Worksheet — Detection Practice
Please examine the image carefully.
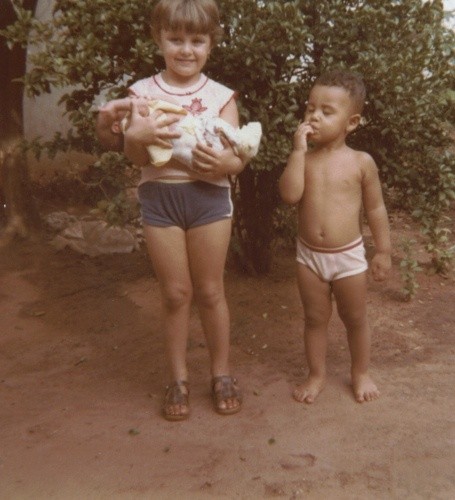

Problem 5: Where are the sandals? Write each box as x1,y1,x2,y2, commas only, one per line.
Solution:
211,375,244,415
163,379,190,420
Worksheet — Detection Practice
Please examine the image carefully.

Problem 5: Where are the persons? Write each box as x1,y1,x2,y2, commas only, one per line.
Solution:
277,68,394,405
124,0,245,423
95,86,262,183
